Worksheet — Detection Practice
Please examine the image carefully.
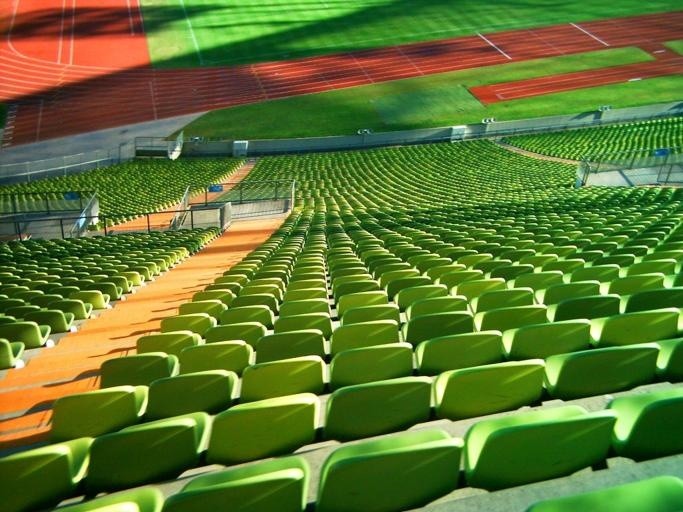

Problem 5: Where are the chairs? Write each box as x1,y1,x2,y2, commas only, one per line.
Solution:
0,114,683,511
